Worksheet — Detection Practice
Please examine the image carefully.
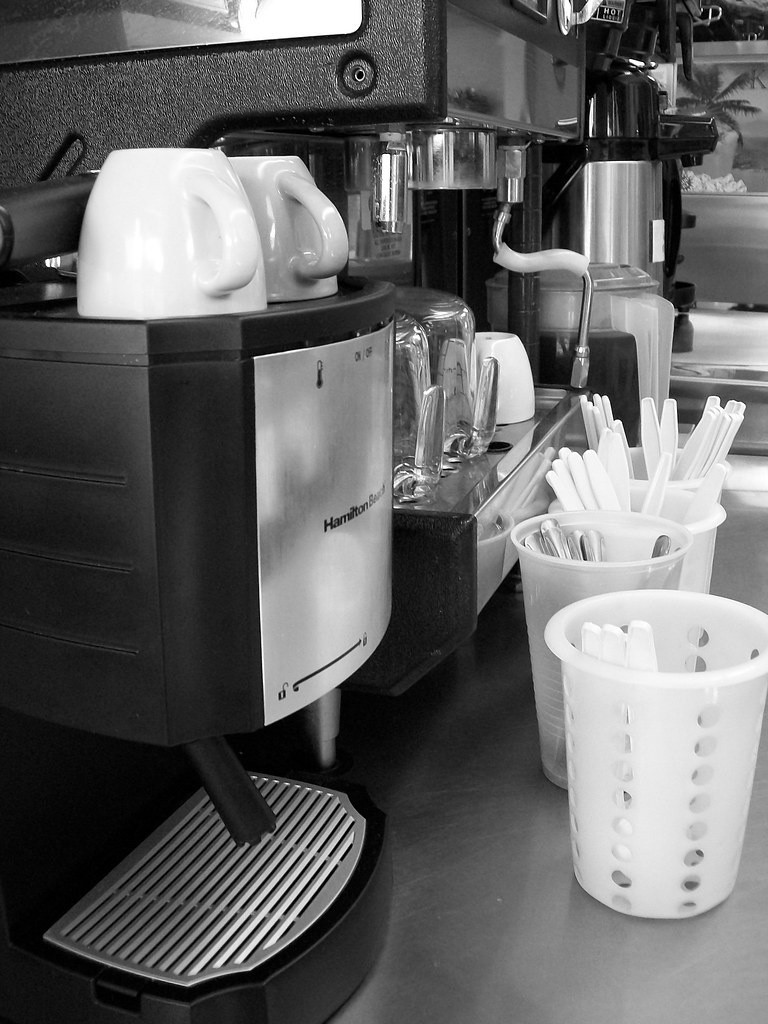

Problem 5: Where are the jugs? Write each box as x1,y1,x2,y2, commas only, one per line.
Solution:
486,263,675,447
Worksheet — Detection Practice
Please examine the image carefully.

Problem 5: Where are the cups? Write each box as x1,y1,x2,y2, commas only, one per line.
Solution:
76,148,268,323
600,446,732,508
393,311,445,503
228,156,349,303
546,488,729,594
510,509,694,791
473,331,536,424
544,589,768,920
395,289,498,463
